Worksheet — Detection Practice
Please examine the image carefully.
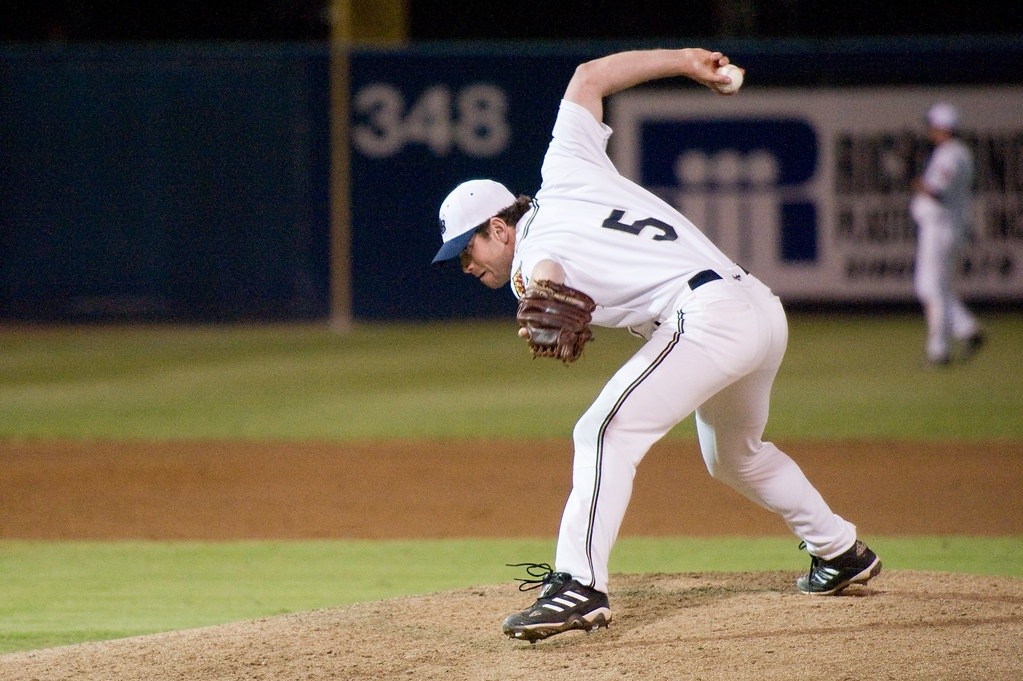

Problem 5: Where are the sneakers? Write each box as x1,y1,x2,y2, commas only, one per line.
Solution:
796,539,882,596
502,562,612,639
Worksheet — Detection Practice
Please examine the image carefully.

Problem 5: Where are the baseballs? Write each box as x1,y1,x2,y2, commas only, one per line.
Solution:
716,64,744,92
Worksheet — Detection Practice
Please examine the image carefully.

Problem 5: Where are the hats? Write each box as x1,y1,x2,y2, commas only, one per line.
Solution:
431,178,517,265
925,104,960,130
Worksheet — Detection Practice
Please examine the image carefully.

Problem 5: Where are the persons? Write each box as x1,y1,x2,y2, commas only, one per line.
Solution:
432,48,883,644
910,103,987,370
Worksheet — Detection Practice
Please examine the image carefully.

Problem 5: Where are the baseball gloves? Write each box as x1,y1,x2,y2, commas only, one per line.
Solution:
517,278,598,364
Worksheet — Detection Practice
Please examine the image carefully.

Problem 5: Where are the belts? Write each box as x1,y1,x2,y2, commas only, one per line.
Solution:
654,263,749,326
